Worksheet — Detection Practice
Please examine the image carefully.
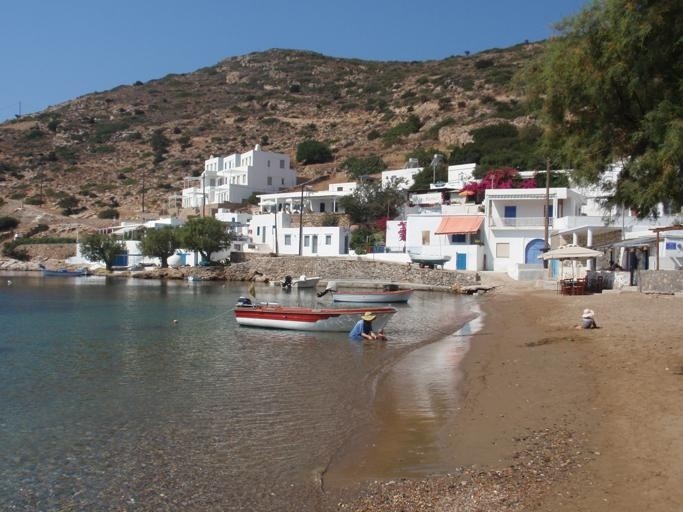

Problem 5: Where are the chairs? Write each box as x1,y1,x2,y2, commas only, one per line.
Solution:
560,276,603,295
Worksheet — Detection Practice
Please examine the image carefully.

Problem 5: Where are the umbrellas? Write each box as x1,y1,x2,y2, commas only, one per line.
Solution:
536,246,576,279
543,244,603,278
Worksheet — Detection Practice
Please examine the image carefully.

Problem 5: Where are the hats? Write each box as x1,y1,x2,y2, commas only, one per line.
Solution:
581,309,594,318
360,312,375,321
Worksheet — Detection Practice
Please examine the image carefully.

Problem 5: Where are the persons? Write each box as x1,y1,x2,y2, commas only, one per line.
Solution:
348,311,376,344
575,308,600,331
629,245,649,286
608,260,621,273
373,329,389,341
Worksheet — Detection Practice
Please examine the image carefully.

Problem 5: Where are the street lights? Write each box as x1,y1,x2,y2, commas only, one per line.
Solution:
298,183,313,257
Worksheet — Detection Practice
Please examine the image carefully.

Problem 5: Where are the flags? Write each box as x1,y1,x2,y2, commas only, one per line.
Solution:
248,281,255,297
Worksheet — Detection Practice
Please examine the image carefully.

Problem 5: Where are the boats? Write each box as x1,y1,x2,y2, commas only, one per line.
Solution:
409,250,451,270
41,268,93,277
184,274,218,282
233,294,397,337
268,275,321,289
317,281,415,305
